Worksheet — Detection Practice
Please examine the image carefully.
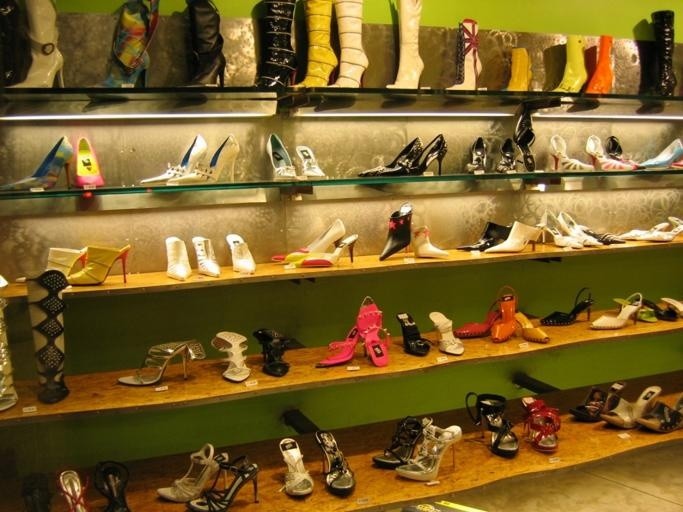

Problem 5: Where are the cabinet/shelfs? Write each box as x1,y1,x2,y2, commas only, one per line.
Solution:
0,82,683,510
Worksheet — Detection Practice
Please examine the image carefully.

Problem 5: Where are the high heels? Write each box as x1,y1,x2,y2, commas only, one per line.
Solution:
16,244,131,286
453,285,550,343
396,312,430,355
59,470,90,511
357,134,447,177
620,217,683,242
157,443,258,511
586,135,645,172
265,134,325,182
23,473,53,511
521,397,562,450
466,111,536,174
142,135,241,188
316,296,391,368
254,328,289,377
549,135,594,171
380,203,449,262
226,235,256,274
429,312,465,356
314,431,355,494
373,415,463,482
2,135,104,192
192,237,220,277
641,139,682,167
465,391,518,459
455,221,541,252
271,219,359,268
591,293,682,329
166,237,192,281
94,461,130,511
541,287,595,326
568,380,683,433
118,339,206,385
279,438,313,496
541,211,625,249
211,332,250,382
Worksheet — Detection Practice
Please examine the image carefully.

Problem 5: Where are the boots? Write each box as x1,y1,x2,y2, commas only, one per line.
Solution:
252,0,369,88
647,10,678,95
552,35,613,94
507,47,533,92
94,0,160,89
386,1,425,89
445,18,483,90
178,0,225,87
0,1,65,87
